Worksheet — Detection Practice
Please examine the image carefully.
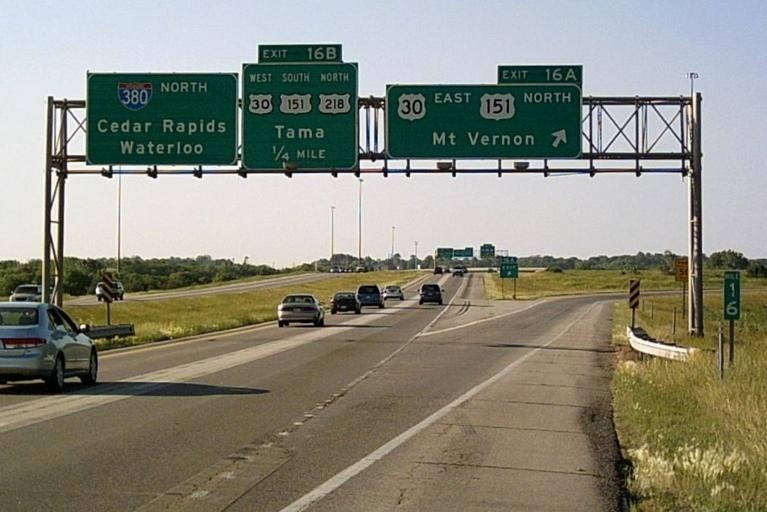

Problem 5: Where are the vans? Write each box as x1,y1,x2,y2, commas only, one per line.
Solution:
357,284,385,309
416,283,444,305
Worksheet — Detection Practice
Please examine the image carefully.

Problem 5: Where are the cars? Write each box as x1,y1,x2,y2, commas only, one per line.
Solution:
329,264,369,273
382,284,405,301
0,300,98,389
434,265,467,277
276,294,324,327
331,292,360,316
486,269,497,273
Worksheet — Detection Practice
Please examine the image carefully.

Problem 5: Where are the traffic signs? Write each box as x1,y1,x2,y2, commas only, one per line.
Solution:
84,71,240,167
436,248,452,258
257,43,342,64
240,64,358,170
385,84,582,157
454,250,465,256
500,263,518,278
503,257,518,262
496,63,582,86
674,257,689,281
480,246,494,258
464,247,472,256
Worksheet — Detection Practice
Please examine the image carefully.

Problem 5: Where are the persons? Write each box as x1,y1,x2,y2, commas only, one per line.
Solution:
18,310,35,325
53,317,66,332
304,298,312,303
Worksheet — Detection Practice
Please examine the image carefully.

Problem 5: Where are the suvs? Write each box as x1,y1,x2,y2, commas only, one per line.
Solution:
94,280,124,303
9,282,54,305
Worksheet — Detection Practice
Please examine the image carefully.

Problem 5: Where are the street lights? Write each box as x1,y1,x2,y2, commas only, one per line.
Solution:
357,178,364,265
390,226,396,259
331,206,337,264
414,241,418,269
